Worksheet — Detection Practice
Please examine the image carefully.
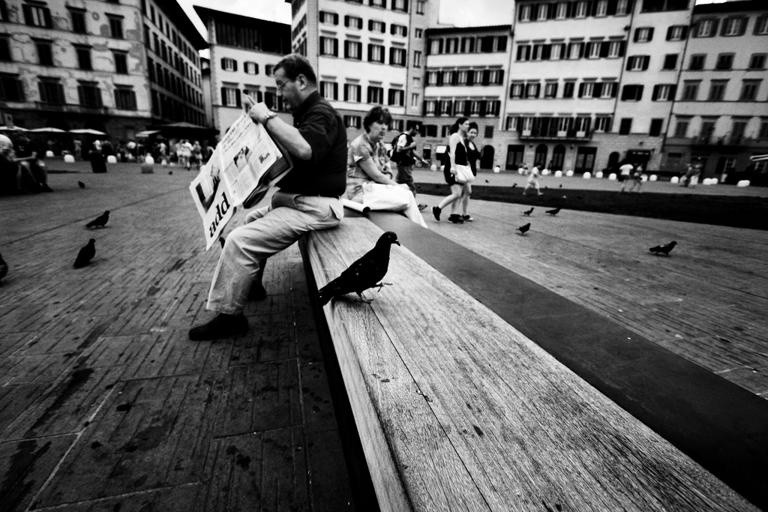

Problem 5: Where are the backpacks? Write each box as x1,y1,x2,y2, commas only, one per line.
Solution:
386,133,417,163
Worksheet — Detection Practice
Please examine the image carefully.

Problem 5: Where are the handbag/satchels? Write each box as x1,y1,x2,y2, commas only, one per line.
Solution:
453,163,475,184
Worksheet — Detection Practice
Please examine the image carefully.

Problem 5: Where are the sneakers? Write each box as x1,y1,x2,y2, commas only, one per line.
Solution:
417,203,471,224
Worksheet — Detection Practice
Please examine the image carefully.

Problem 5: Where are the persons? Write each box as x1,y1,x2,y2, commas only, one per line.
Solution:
629,162,644,193
521,162,544,196
618,161,634,195
187,52,346,346
344,104,429,229
431,115,469,226
1,106,204,189
462,121,486,224
389,120,431,213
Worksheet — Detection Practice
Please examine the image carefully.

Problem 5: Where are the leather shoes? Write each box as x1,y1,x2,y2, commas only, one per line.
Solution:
188,310,249,342
239,285,268,305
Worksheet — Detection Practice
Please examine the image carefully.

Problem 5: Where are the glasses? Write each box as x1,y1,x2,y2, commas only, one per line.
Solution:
276,79,293,92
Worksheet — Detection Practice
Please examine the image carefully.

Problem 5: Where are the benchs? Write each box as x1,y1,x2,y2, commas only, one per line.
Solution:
295,212,766,511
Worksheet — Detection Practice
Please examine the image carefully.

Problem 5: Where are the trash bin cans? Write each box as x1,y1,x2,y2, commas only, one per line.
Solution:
89,149,107,173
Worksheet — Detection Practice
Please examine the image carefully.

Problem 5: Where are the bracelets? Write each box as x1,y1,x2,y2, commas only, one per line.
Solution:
383,170,393,180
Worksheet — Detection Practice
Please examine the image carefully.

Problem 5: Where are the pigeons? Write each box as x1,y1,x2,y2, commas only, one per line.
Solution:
73,238,96,269
649,241,678,255
524,207,534,216
545,208,561,217
318,231,400,306
517,223,530,235
78,180,85,189
85,210,111,229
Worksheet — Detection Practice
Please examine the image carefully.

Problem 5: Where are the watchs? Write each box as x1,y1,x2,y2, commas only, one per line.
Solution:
262,110,278,127
